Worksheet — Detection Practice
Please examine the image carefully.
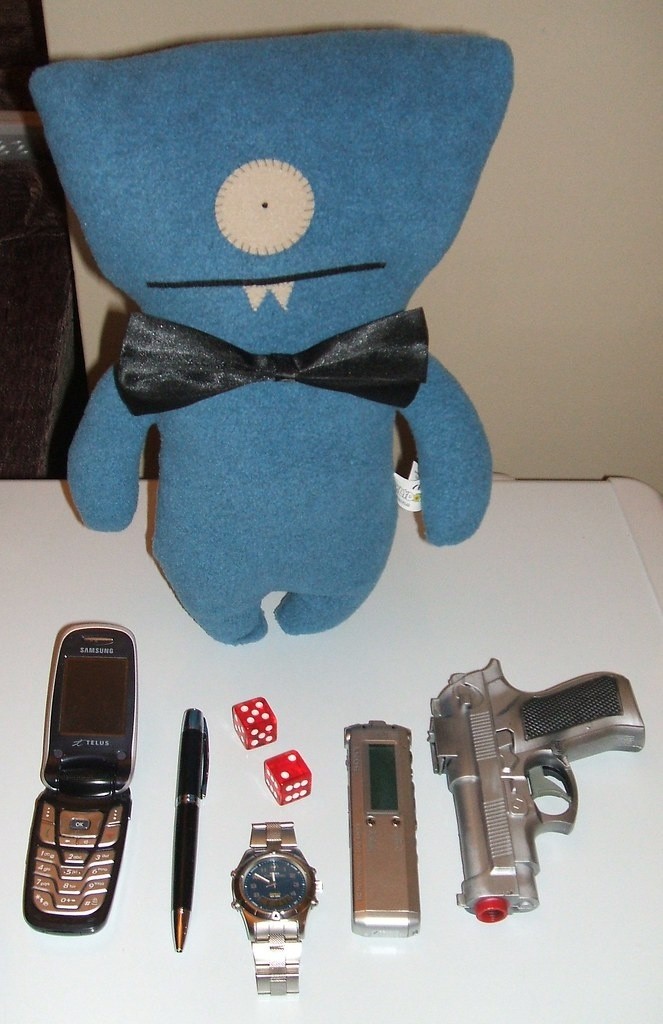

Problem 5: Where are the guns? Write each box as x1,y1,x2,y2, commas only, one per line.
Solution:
426,656,647,925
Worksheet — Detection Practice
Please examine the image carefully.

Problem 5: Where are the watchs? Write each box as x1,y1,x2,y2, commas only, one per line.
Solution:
231,822,324,997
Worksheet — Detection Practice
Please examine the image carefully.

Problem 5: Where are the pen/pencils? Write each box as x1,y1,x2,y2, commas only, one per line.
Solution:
172,710,212,954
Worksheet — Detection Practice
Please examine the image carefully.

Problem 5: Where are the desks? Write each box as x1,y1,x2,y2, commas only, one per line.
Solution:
0,471,663,1024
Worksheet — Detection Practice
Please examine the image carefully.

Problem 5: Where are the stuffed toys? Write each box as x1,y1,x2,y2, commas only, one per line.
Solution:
27,29,515,644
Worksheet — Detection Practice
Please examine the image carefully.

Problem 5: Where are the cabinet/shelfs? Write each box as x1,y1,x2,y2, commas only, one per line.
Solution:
0,0,86,474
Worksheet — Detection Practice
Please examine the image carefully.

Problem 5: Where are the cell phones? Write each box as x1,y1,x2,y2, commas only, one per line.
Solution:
22,623,137,935
